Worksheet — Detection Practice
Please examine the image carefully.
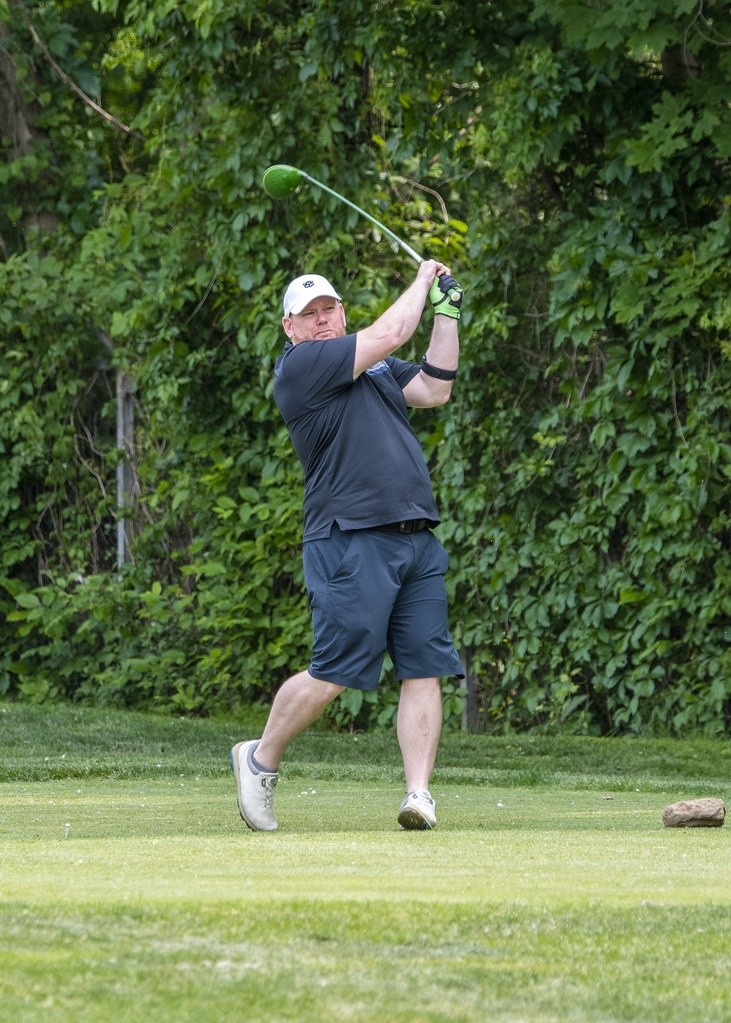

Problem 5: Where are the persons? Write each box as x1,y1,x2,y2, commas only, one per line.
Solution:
230,259,463,828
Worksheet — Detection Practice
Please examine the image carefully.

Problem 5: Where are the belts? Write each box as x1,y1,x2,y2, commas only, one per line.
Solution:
369,519,426,534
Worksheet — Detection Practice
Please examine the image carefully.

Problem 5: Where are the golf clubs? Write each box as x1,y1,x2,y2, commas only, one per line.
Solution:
261,163,462,303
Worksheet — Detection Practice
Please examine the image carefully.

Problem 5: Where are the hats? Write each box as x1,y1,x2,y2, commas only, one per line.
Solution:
283,274,342,317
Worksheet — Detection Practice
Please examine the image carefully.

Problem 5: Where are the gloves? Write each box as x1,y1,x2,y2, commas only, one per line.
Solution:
430,273,464,320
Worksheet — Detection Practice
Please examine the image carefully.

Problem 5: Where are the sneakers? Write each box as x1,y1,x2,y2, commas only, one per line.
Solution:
230,740,278,832
398,789,437,829
